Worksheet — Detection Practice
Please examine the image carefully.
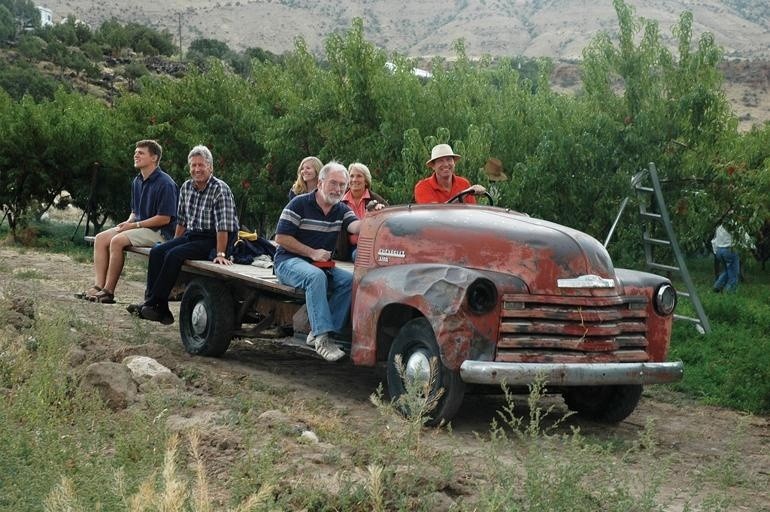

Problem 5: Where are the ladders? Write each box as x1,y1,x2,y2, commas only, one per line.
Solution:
634,162,711,335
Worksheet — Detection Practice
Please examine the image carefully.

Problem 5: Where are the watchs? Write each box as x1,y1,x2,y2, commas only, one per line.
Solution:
215,252,226,257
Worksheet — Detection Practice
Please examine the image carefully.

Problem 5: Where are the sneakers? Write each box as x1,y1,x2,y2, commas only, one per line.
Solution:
126,303,175,325
305,335,347,363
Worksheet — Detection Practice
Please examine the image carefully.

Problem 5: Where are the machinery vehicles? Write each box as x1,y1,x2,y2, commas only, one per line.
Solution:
83,191,684,427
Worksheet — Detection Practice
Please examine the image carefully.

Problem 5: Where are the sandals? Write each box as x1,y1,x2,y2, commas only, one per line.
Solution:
74,286,116,303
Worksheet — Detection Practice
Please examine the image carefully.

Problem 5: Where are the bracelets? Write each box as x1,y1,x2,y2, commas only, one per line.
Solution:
136,221,140,228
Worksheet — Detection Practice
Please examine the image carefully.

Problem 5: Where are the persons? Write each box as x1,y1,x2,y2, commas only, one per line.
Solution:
335,163,389,262
413,144,486,204
711,210,757,296
274,162,385,362
482,158,509,201
290,156,323,201
75,140,180,303
126,144,240,325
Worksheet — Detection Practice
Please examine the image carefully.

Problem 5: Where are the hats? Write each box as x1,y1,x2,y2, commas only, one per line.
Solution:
425,143,462,167
479,157,507,182
